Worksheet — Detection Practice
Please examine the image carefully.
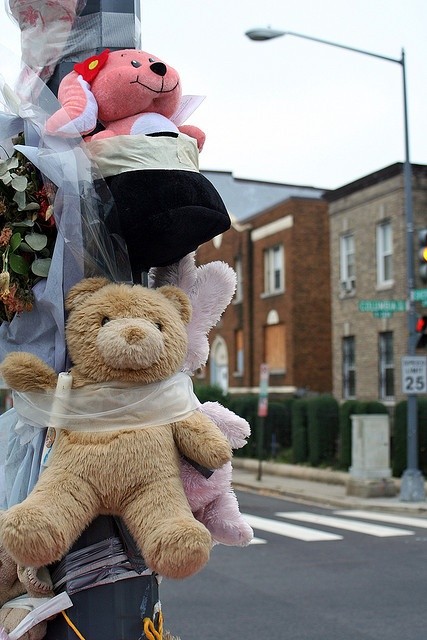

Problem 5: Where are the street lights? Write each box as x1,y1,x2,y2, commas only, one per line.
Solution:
246,28,427,503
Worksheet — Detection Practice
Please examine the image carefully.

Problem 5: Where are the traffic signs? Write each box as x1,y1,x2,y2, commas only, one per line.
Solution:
402,354,427,394
409,289,427,308
358,299,409,318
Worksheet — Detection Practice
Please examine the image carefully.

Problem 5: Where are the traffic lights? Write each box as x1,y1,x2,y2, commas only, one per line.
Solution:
417,229,427,281
416,314,427,349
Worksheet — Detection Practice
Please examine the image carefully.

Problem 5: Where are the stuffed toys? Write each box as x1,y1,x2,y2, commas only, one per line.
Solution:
0,544,53,640
44,49,232,266
0,276,234,579
144,249,255,548
